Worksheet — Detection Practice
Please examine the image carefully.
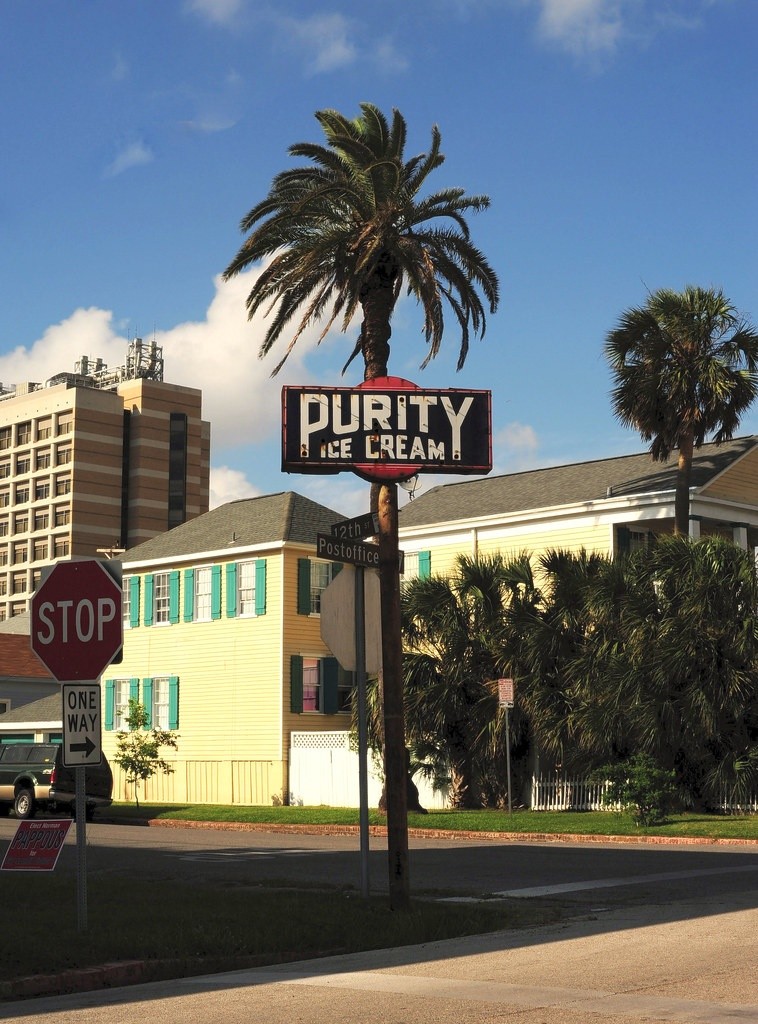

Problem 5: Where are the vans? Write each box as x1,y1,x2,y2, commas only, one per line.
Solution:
0,743,113,822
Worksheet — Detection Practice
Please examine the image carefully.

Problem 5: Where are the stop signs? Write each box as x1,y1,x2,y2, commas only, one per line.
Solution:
29,557,124,683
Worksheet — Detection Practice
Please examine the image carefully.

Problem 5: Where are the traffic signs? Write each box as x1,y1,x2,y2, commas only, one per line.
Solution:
63,680,102,767
